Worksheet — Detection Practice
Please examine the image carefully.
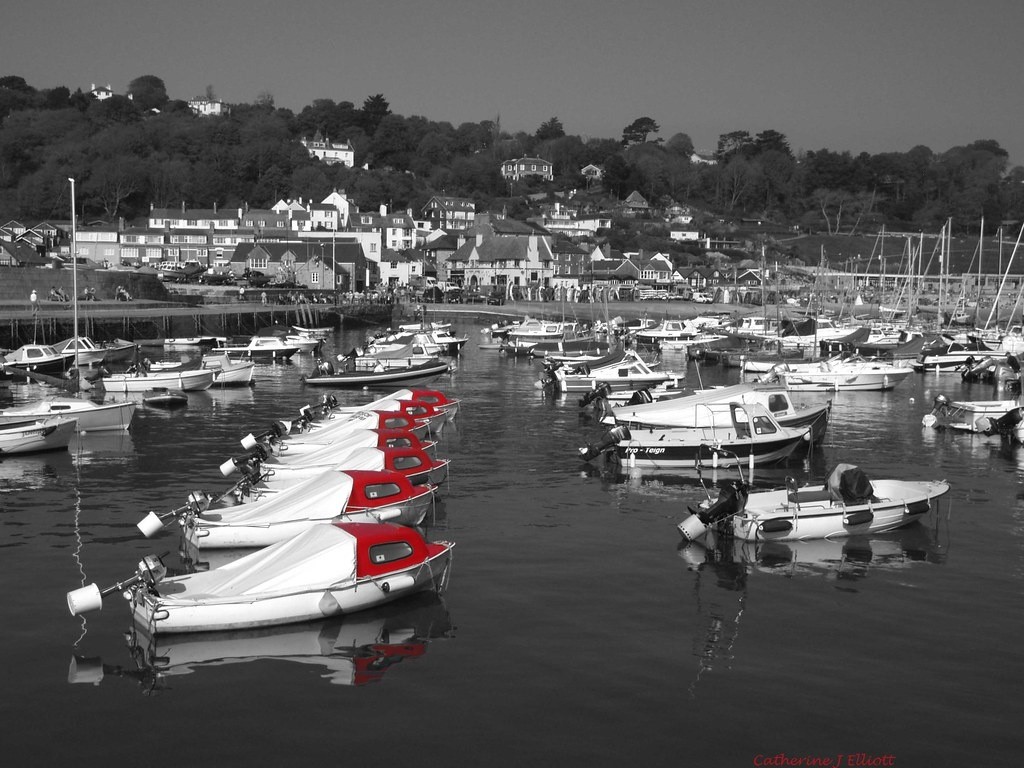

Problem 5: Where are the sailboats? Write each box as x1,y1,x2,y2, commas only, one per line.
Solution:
38,214,1024,407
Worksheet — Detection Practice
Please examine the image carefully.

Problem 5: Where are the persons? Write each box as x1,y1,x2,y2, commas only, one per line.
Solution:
48,284,133,304
236,278,413,306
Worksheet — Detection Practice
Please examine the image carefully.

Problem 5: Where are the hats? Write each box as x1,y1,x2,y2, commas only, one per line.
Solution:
1005,352,1010,356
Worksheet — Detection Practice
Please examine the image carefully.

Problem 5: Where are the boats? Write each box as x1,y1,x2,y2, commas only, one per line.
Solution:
611,382,832,446
64,521,457,638
134,467,438,551
218,386,463,482
916,340,1011,373
922,393,1024,433
578,400,811,467
1,342,74,379
677,442,951,543
0,394,139,432
1,414,77,453
974,406,1024,443
742,351,914,391
